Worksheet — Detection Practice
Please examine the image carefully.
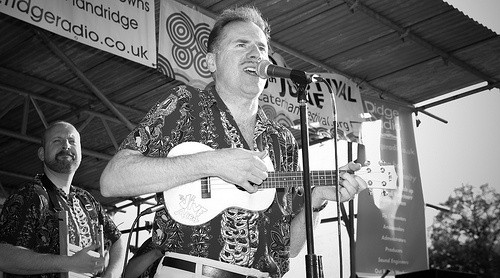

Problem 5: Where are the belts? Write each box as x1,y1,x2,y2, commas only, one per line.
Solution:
161,257,257,278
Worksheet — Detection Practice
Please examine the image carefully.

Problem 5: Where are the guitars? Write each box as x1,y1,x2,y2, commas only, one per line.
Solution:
163,141,399,226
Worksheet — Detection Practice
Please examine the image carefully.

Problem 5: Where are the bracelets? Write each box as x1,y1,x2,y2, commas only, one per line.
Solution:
311,186,328,212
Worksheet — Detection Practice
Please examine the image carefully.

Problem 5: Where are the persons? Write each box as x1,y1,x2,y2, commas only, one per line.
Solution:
0,122,126,278
100,7,368,278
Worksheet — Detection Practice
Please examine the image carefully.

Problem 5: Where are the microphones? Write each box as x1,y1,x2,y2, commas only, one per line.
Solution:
256,60,324,84
